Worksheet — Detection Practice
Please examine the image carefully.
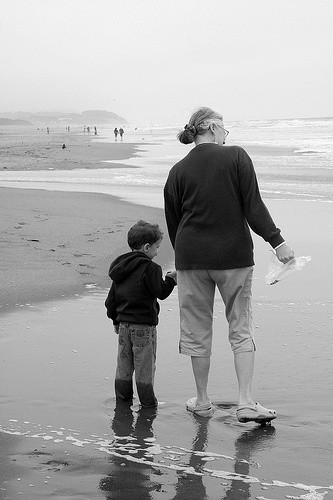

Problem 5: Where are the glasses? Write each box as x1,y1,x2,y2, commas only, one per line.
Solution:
217,125,229,137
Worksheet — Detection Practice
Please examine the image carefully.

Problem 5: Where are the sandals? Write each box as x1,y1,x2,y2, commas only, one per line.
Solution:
235,402,277,423
186,397,212,417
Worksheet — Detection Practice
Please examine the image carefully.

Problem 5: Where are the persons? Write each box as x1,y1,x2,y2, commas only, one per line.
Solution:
114,127,124,137
163,107,296,424
104,219,178,408
83,125,97,132
